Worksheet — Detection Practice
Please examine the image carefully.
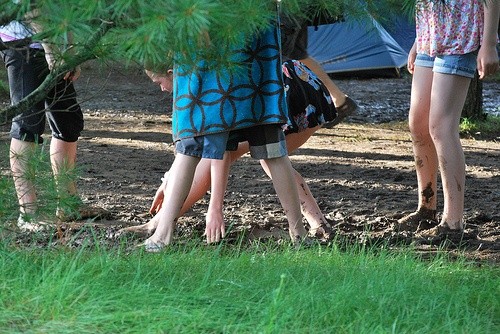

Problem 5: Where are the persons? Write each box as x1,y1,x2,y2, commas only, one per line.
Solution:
299,56,358,129
0,10,112,234
116,57,337,245
144,0,306,250
395,0,500,243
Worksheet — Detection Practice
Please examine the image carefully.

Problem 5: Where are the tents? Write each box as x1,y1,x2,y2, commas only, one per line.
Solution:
307,0,416,79
